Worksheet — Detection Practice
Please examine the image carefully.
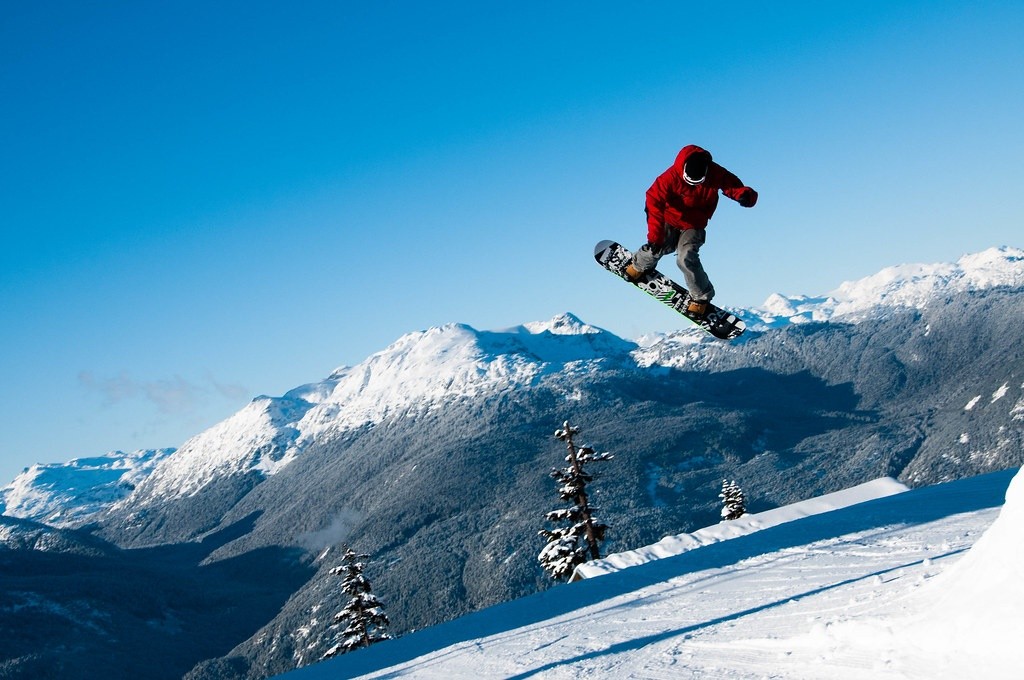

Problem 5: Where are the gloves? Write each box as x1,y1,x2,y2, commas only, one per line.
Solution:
739,191,750,207
650,243,660,255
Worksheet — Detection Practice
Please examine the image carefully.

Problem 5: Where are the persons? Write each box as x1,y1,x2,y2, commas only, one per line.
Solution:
622,143,759,319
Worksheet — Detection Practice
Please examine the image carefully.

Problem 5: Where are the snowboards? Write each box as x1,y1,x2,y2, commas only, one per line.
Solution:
595,238,747,340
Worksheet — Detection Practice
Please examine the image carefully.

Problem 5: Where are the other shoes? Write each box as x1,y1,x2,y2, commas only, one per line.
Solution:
624,265,642,280
688,300,708,315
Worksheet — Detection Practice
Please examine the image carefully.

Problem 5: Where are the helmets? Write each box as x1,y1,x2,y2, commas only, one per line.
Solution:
683,153,710,185
